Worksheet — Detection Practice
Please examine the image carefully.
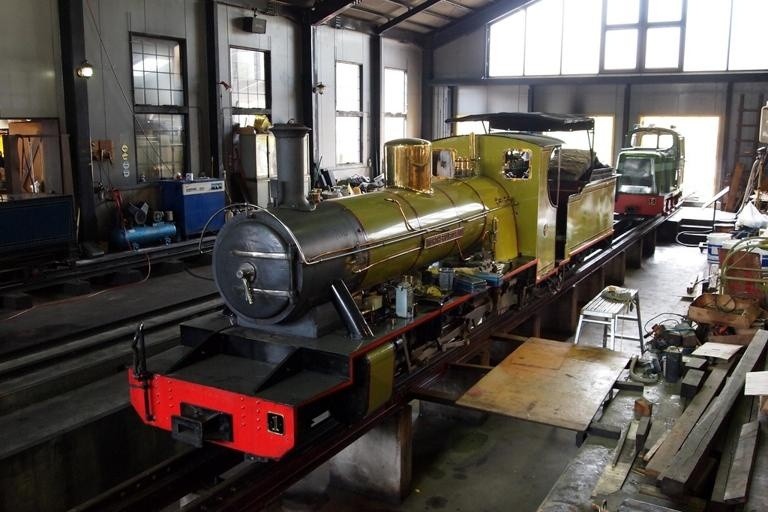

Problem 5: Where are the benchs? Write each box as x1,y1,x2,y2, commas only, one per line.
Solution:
573,284,644,355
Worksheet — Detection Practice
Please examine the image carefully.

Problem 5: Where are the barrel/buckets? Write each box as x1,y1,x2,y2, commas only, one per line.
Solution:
755,248,768,270
396,284,413,317
439,267,454,290
706,232,731,264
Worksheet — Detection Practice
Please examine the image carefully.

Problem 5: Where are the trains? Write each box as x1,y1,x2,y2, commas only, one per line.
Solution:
128,111,685,480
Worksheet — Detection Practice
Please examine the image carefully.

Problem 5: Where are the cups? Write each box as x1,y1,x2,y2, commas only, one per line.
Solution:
440,267,455,292
186,173,193,181
